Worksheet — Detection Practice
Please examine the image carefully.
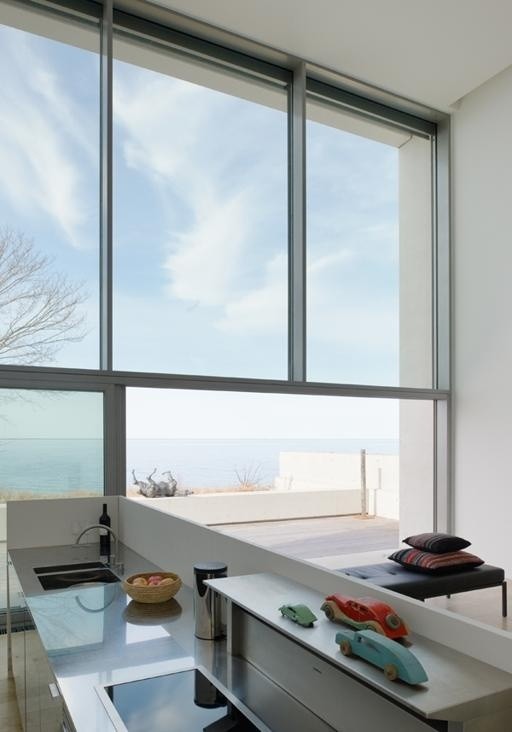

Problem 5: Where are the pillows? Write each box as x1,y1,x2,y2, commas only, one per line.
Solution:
387,529,482,575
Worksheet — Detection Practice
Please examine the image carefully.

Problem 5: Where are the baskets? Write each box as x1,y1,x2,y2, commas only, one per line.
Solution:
124,573,182,603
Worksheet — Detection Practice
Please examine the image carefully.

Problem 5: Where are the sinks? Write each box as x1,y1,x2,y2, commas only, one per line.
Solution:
32,561,110,575
36,567,122,591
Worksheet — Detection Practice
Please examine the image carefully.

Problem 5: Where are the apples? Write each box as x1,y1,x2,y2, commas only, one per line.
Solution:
132,575,174,586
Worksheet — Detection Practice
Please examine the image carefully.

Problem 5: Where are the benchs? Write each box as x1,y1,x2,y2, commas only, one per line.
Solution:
332,558,507,620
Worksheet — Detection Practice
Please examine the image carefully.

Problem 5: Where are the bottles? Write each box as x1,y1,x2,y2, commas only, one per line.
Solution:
192,561,229,642
99,503,111,555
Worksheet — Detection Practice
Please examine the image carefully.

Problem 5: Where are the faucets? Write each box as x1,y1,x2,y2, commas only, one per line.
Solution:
75,585,121,613
74,523,119,566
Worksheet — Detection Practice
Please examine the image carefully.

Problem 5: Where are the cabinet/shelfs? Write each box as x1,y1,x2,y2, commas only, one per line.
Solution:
6,552,63,731
